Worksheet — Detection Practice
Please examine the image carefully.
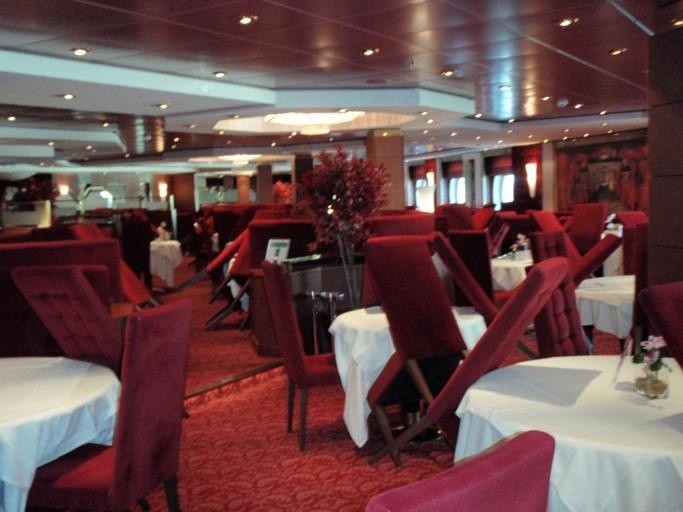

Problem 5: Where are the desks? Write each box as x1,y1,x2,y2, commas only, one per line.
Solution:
454,356,683,511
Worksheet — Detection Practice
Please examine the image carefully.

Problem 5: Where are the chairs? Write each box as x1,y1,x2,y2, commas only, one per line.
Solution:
0,208,192,512
193,204,682,469
364,430,555,511
